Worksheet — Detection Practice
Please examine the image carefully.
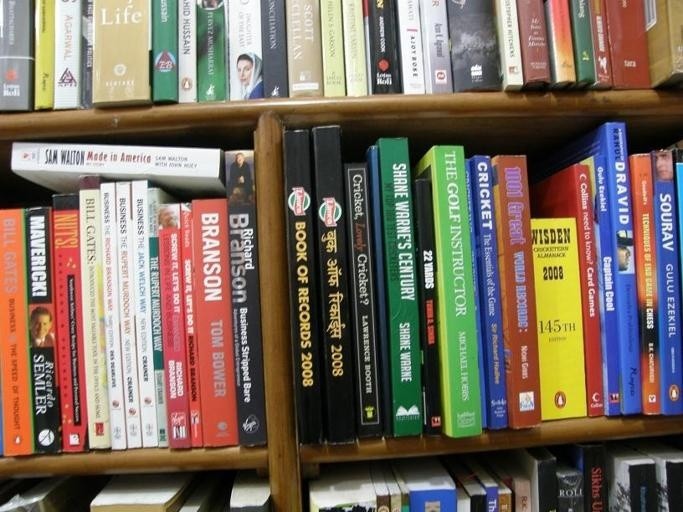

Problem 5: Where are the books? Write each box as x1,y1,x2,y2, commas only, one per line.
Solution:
0,0,683,113
309,436,683,512
1,142,267,456
0,474,272,512
283,121,683,446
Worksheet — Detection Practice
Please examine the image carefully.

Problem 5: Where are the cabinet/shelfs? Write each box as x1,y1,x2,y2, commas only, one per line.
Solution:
0,86,683,512
